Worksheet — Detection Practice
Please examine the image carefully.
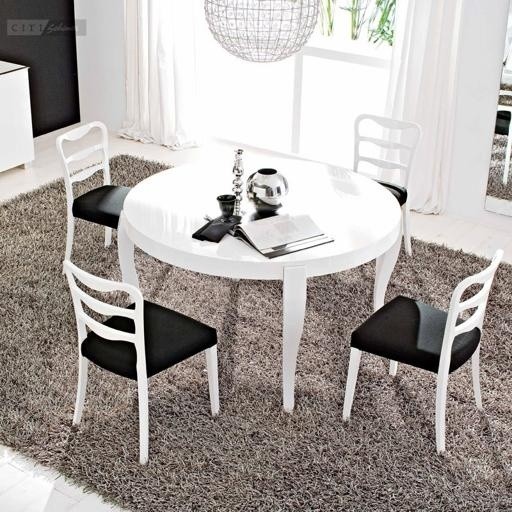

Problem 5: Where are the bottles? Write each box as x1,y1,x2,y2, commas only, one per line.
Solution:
246,168,289,212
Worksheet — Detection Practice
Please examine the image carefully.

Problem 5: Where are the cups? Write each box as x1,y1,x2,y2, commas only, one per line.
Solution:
216,194,236,222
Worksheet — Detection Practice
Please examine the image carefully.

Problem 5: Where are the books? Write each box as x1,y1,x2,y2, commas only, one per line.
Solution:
203,204,336,259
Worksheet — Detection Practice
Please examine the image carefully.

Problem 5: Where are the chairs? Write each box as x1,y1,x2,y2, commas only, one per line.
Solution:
353,112,421,259
62,261,221,465
343,249,505,454
56,118,139,277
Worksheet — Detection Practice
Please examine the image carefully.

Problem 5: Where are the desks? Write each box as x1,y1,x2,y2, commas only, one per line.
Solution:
117,147,403,414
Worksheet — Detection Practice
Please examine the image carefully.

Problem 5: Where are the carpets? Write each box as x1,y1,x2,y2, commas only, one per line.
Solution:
0,150,512,510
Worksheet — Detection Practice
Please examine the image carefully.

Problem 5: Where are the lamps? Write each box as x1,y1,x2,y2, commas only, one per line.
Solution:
204,1,320,64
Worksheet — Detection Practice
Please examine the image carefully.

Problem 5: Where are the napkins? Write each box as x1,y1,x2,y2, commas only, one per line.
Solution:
191,215,242,243
